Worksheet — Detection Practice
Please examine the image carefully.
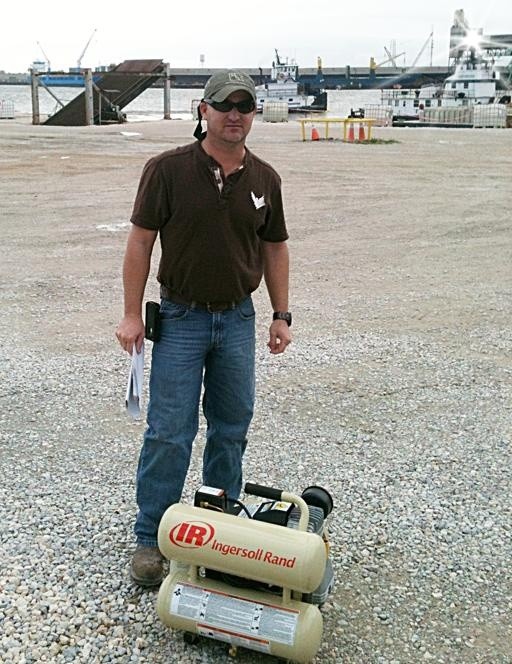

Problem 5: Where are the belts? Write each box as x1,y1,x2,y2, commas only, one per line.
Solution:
160,285,240,313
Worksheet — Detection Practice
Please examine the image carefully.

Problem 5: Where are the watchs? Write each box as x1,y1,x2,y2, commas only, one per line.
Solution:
273,312,291,327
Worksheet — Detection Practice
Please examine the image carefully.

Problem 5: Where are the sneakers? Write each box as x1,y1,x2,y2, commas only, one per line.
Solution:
128,544,164,586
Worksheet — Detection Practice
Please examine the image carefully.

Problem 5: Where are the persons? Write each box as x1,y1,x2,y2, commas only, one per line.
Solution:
116,70,292,587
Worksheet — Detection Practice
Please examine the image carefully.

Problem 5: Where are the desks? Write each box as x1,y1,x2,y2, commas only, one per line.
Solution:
296,117,376,145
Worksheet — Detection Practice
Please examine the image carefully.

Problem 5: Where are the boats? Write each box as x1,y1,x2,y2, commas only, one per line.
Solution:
255,57,301,112
330,46,512,127
33,29,116,86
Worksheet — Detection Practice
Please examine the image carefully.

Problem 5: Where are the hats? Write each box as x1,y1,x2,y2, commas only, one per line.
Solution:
204,68,256,105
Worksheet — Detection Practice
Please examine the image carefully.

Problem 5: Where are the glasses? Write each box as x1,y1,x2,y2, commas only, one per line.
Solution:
203,99,256,113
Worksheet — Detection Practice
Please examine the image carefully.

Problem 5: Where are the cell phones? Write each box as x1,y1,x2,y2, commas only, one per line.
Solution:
147,301,161,337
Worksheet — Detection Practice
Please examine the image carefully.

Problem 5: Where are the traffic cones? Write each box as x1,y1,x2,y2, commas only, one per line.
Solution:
310,122,319,141
347,116,367,142
